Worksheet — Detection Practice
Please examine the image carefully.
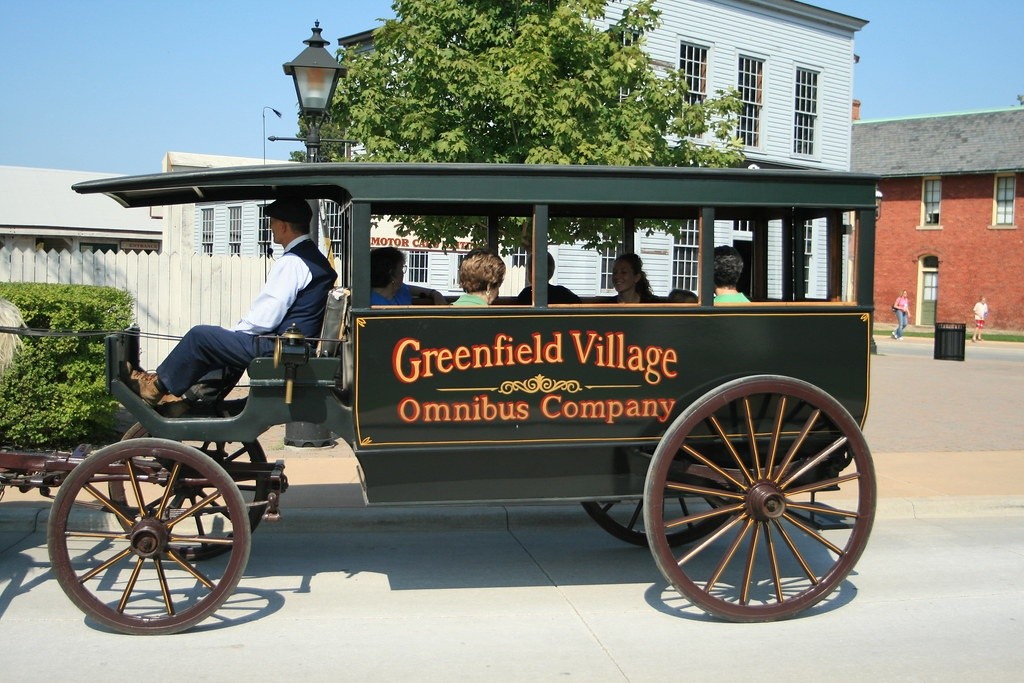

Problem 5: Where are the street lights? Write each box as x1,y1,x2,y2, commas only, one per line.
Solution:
281,16,351,253
260,105,283,286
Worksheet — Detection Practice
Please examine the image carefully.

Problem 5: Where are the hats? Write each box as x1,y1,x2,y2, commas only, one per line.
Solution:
264,198,313,223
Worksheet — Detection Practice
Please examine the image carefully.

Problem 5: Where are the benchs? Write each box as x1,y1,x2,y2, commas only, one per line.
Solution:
249,284,350,379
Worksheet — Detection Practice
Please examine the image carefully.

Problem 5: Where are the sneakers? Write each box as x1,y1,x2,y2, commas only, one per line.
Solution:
121,360,166,409
156,394,192,417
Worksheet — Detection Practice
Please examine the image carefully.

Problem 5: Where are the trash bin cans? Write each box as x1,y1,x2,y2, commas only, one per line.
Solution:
283,420,337,448
934,322,965,362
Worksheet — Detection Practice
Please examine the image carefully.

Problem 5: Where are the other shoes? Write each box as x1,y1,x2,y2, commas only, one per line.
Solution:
892,332,898,338
978,338,982,340
972,339,977,343
899,337,904,341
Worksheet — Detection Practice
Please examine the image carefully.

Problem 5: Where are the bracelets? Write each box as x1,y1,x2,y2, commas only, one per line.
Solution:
428,290,435,297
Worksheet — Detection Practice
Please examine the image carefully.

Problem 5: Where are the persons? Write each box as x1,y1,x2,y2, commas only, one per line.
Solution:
370,247,447,306
713,245,751,303
667,289,697,303
972,296,989,341
891,289,911,339
598,253,666,303
120,199,339,418
448,247,506,305
517,252,582,305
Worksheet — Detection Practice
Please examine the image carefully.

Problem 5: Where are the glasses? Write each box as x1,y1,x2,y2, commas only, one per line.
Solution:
401,266,407,274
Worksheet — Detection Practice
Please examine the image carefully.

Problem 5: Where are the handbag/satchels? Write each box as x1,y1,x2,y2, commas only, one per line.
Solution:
892,295,902,312
